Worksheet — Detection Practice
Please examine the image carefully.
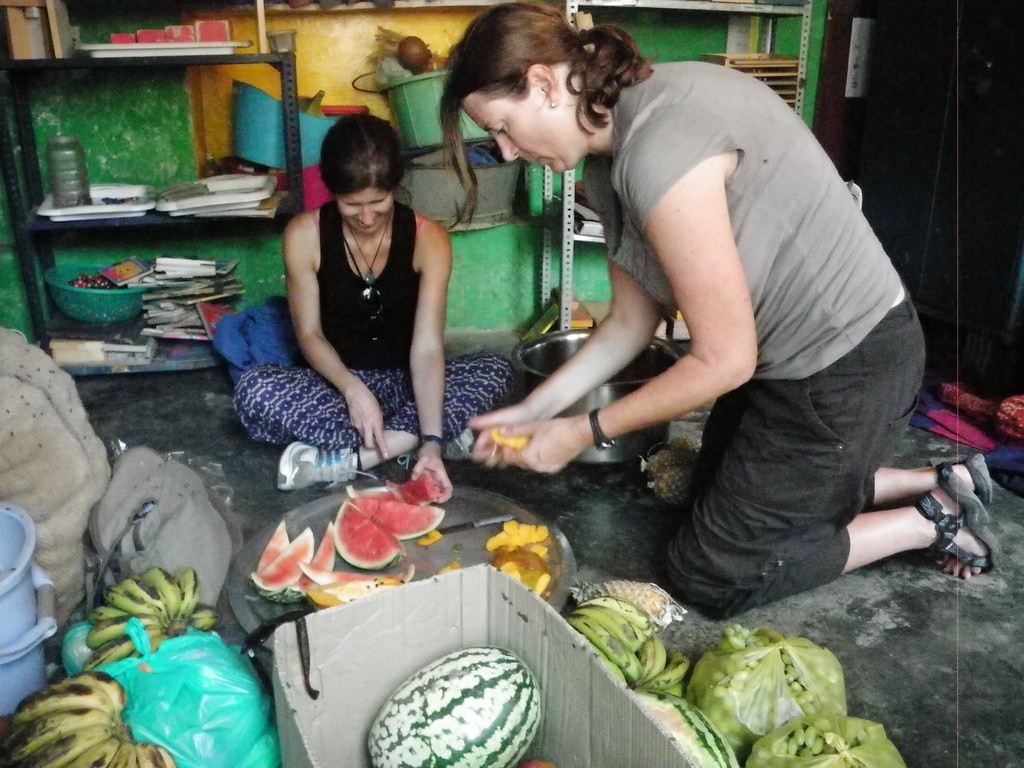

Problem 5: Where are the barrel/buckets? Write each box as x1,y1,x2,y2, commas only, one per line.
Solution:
352,66,496,148
0,501,58,719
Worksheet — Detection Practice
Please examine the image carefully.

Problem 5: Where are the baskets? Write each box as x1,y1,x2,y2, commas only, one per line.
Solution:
44,263,149,324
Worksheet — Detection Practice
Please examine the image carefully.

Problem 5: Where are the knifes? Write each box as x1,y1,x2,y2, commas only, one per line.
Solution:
437,513,515,535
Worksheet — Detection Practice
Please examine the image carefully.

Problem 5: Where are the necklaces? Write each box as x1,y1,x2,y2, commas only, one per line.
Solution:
346,216,391,286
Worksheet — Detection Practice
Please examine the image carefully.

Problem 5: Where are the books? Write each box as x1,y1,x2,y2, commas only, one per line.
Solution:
48,256,244,366
194,191,290,220
546,180,606,238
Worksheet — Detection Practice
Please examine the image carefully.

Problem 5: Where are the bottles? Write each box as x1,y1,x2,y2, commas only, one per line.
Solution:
47,135,92,208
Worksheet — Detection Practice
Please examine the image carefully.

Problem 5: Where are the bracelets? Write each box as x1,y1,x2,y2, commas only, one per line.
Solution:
421,435,447,454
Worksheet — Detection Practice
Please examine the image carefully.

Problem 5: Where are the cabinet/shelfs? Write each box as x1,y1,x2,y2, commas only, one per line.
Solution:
0,53,304,375
834,1,1024,346
539,0,813,341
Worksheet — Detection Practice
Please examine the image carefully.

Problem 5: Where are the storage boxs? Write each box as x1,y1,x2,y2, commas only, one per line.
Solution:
377,68,498,152
228,78,349,172
230,158,333,222
272,562,697,768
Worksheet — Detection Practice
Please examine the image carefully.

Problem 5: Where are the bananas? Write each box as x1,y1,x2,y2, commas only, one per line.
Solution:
85,567,220,667
0,670,178,768
564,596,689,700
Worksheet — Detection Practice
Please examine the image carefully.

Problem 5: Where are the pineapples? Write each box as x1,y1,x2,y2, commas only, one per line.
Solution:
486,521,549,552
640,438,701,472
646,468,693,502
569,579,668,620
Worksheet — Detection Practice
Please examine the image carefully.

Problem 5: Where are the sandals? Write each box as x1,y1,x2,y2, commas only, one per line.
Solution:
914,454,1000,576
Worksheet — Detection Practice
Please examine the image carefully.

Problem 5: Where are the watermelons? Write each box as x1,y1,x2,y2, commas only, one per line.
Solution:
249,475,446,601
368,645,545,767
631,688,739,768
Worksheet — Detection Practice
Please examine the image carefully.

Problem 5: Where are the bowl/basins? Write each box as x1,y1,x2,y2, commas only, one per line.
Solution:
392,160,522,233
512,328,688,466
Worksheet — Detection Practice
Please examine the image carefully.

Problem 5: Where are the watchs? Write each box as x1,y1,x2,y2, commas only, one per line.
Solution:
589,408,615,451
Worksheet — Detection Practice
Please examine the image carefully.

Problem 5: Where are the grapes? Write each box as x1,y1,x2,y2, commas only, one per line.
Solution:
688,621,905,768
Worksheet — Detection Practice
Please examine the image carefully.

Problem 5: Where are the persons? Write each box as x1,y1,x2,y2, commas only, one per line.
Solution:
438,1,999,622
232,113,512,504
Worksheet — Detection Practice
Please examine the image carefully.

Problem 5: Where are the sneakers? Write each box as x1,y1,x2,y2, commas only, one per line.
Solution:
398,428,475,470
277,442,378,491
